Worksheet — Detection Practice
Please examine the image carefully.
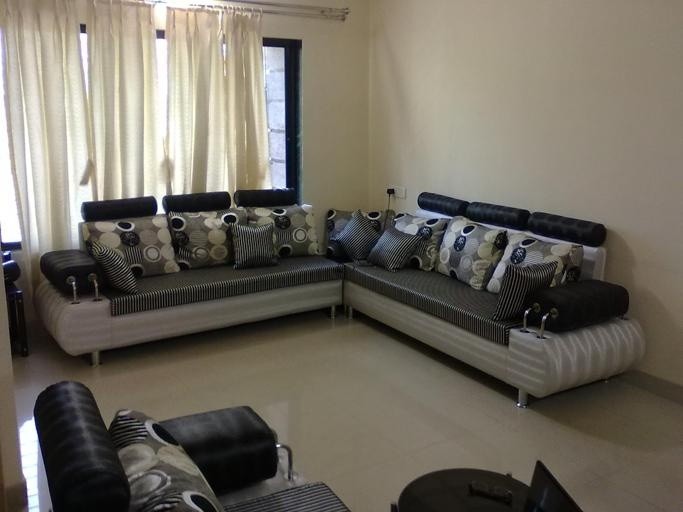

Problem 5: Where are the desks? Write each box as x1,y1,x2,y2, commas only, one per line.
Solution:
389,470,530,511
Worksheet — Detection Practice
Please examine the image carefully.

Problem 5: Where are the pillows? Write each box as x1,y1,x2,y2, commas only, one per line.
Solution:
328,210,584,322
107,410,226,512
80,203,321,296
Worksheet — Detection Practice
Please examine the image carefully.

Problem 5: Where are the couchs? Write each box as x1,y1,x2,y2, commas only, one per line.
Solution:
342,193,645,410
33,188,343,366
17,380,349,511
